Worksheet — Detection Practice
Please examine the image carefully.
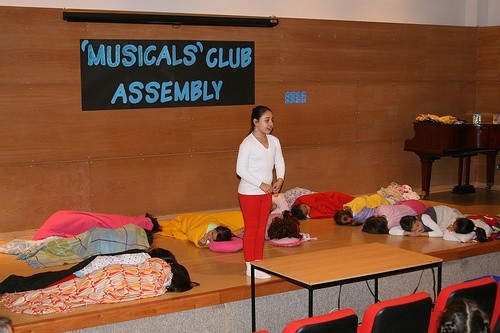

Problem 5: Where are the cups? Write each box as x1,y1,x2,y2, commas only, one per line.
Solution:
493,113,500,124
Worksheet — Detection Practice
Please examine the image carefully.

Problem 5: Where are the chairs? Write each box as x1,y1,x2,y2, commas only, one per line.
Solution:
282,276,500,333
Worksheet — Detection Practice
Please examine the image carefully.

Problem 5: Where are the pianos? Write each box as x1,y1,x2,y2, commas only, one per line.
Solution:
412,119,496,201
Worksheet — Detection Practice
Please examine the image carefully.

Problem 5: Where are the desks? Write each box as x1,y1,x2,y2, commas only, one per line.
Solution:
250,241,444,333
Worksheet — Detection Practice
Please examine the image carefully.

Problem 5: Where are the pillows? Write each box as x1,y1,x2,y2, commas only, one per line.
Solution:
268,233,310,247
208,236,243,253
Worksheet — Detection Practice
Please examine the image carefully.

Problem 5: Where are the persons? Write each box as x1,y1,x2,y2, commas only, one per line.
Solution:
270,187,495,243
439,298,491,333
33,210,304,304
235,105,285,279
